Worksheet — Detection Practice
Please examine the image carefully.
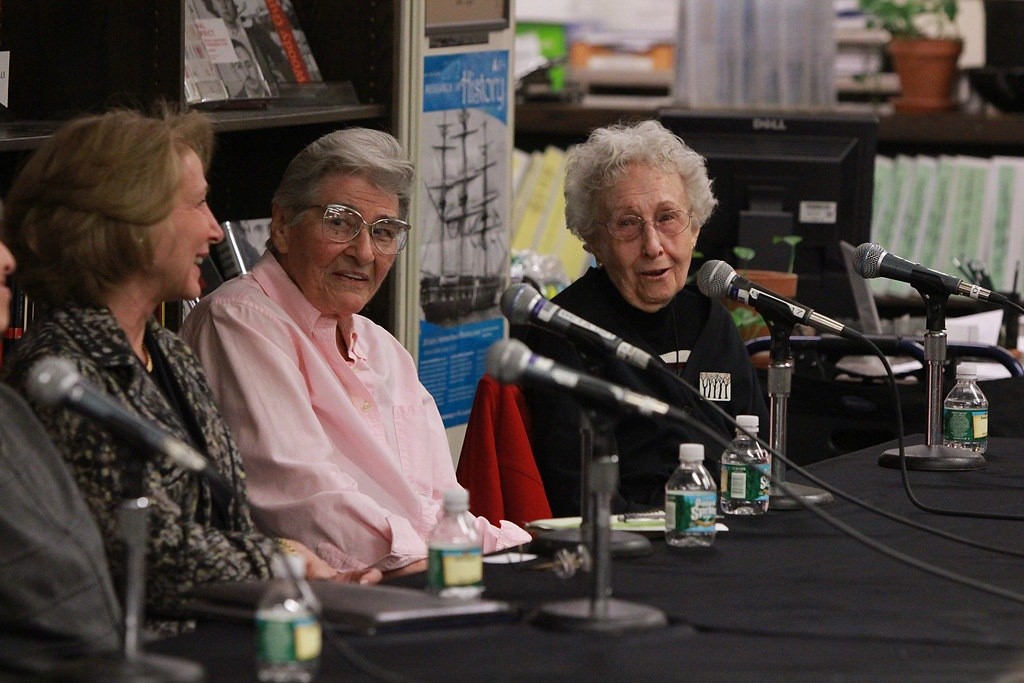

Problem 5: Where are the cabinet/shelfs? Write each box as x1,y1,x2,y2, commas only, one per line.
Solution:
0,0,411,358
512,102,1024,322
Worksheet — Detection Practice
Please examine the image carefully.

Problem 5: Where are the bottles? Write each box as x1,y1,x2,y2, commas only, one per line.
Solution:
252,552,323,683
720,415,771,516
943,364,989,455
664,442,718,548
424,488,486,601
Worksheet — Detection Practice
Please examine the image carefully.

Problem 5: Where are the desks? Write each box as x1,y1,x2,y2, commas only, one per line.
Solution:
143,431,1024,683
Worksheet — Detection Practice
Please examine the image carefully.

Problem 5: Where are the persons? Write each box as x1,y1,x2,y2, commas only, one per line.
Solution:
228,39,270,98
0,104,380,683
511,121,772,516
177,127,531,586
202,0,242,38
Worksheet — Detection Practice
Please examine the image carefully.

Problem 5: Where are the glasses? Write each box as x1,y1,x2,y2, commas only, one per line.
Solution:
297,204,412,256
594,206,694,242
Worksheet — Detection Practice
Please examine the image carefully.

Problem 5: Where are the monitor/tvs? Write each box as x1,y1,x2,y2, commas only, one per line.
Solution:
659,106,877,322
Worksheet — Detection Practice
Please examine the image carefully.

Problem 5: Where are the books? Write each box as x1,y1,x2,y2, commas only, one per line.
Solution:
183,0,323,111
182,217,273,323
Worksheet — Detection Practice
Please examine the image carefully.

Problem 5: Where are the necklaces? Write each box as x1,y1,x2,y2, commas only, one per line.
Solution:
142,344,153,373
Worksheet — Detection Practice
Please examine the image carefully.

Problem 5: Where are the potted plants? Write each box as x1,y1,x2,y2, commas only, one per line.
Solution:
857,0,965,117
689,236,802,364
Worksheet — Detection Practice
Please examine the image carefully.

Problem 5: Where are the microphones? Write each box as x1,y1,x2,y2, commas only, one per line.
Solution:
698,260,862,339
500,282,664,371
24,357,223,485
485,337,686,417
851,243,1009,306
